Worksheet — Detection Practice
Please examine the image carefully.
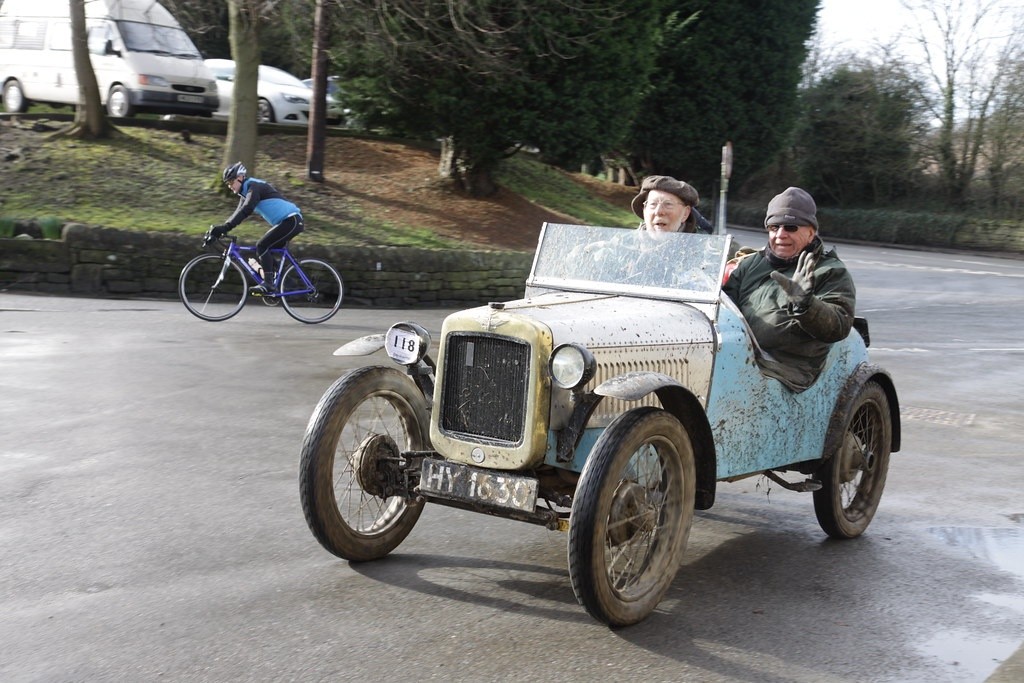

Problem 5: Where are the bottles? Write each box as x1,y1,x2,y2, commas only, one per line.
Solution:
247,257,262,273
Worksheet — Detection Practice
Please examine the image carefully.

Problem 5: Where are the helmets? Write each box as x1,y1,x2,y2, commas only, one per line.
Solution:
223,162,247,182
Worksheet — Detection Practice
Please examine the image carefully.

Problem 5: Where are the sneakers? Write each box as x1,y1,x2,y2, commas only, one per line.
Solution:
248,280,276,294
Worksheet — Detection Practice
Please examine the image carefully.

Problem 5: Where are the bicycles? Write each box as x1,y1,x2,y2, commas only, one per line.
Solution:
178,225,344,324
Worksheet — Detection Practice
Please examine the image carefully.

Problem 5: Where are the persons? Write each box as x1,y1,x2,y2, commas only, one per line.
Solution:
205,161,305,296
563,174,717,291
720,187,857,394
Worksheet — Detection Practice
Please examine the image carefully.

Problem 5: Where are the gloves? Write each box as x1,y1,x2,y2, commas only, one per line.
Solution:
769,249,817,310
211,226,228,238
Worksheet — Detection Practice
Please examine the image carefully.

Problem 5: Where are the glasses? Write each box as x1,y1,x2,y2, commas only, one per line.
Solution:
767,225,807,232
643,199,687,209
227,177,239,185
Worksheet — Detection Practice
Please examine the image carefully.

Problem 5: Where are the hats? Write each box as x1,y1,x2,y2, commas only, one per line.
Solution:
631,175,698,220
764,186,819,231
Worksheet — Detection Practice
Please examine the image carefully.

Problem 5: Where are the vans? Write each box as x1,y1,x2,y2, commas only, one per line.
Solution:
0,0,220,118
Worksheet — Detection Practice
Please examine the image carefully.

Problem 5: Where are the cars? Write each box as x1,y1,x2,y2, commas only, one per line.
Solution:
205,59,348,125
299,222,901,627
302,74,406,120
